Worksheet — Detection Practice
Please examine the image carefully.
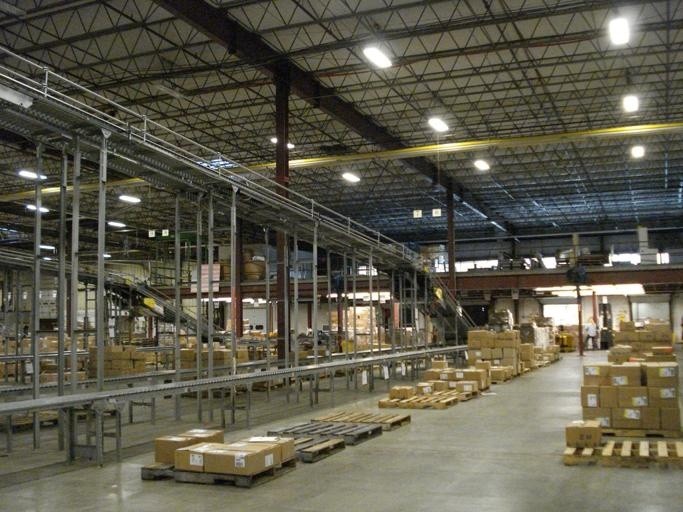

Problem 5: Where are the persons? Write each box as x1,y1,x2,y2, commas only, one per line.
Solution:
583,318,598,349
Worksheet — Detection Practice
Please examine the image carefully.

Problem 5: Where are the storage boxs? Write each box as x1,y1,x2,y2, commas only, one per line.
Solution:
158,427,295,477
39,329,261,393
391,327,561,399
565,318,682,447
